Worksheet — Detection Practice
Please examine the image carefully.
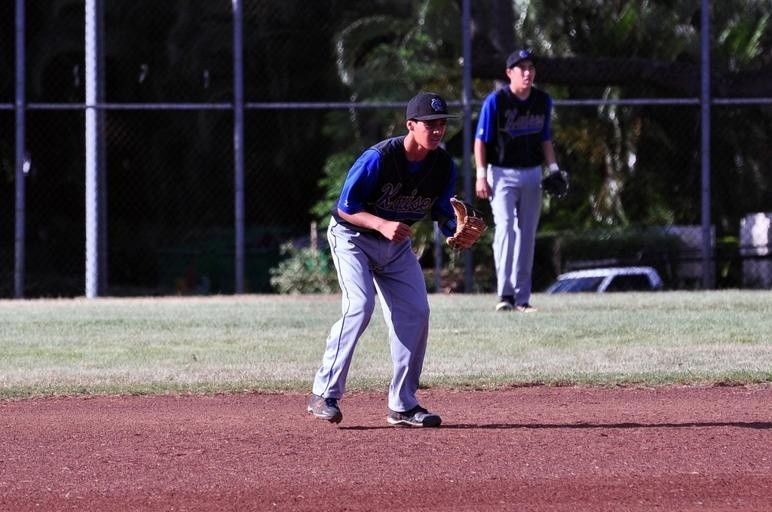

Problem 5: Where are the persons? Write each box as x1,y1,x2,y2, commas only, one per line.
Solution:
305,90,487,430
474,48,570,314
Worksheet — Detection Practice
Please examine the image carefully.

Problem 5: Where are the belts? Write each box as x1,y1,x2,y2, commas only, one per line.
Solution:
360,231,389,242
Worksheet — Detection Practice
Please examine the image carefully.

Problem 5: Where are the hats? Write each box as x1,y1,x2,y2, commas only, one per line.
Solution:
505,49,540,68
407,92,458,120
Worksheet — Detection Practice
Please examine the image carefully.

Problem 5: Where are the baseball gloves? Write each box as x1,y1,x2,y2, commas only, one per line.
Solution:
447,195,486,250
541,171,568,197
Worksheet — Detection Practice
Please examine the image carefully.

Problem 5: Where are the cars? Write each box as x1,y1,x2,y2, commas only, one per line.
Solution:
544,267,673,293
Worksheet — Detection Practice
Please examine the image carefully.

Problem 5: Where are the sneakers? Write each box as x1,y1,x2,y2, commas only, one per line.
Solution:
495,295,515,309
307,393,342,424
516,303,538,313
386,404,442,426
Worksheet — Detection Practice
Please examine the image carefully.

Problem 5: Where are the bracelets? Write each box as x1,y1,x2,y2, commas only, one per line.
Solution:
547,162,559,173
476,166,487,178
